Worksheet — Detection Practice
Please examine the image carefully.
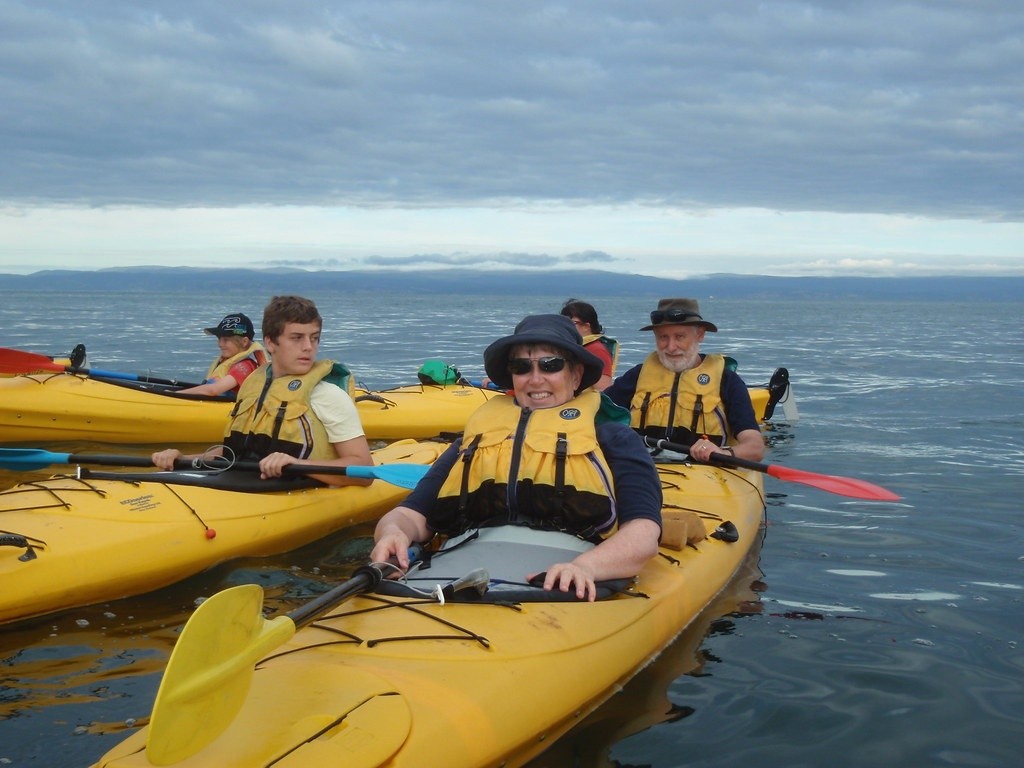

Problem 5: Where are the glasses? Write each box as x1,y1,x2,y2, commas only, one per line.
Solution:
506,356,567,375
650,309,703,325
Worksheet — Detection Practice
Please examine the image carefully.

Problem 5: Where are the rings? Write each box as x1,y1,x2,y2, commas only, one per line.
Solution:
702,446,706,450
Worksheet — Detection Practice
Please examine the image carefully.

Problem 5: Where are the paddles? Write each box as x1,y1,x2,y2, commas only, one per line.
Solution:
640,427,898,502
0,346,208,393
0,448,433,493
145,544,431,767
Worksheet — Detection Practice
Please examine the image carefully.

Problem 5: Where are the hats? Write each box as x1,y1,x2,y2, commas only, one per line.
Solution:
638,298,718,332
203,313,254,340
484,314,604,396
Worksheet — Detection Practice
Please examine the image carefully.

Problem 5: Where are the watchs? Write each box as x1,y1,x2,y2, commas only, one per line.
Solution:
721,445,735,458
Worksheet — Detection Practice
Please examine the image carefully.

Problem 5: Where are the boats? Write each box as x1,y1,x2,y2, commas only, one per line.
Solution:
0,437,451,629
84,442,765,767
0,359,796,464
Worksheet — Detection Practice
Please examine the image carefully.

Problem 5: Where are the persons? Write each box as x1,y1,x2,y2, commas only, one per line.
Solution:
365,314,661,604
162,313,269,400
148,294,375,488
599,297,766,469
481,302,618,395
